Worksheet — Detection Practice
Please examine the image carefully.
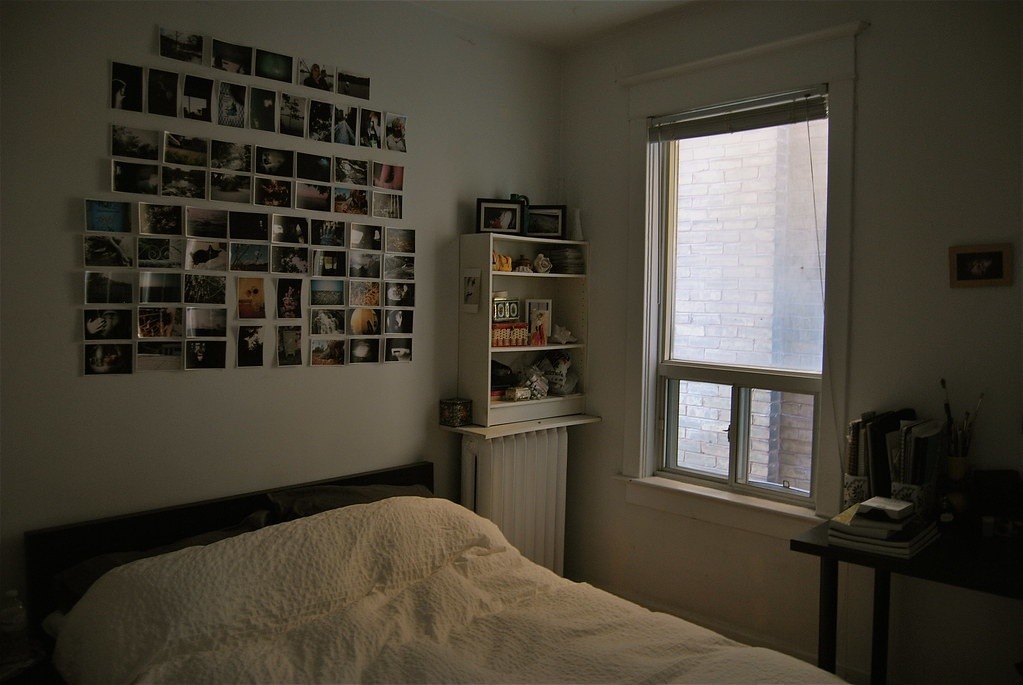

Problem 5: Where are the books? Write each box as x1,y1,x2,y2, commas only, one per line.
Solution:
828,407,950,559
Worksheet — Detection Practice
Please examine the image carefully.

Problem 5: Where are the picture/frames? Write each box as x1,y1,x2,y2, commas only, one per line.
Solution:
476,197,525,237
527,205,568,240
524,299,552,338
949,243,1014,290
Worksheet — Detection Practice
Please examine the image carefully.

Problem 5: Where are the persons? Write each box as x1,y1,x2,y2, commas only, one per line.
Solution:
85,235,131,266
87,310,119,337
490,210,512,229
386,117,406,151
303,63,333,91
343,82,351,95
312,339,344,365
342,189,367,214
361,112,381,145
191,349,208,366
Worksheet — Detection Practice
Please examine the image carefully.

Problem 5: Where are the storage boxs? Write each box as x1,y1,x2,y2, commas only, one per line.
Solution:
439,397,473,427
492,297,520,323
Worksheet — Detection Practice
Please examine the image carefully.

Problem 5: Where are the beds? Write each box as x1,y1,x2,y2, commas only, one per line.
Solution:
27,462,849,685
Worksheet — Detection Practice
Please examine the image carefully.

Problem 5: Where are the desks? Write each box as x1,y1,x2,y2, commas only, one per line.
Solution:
790,520,1023,685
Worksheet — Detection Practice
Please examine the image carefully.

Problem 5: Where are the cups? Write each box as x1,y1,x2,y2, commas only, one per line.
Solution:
948,456,967,478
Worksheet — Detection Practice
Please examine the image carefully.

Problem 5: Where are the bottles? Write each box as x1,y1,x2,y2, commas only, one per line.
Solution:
0,590,34,669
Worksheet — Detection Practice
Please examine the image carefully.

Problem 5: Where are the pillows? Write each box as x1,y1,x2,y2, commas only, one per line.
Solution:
268,485,436,523
23,518,266,649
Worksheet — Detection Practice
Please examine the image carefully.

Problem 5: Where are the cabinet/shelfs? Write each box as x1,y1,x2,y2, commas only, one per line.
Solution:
458,233,590,428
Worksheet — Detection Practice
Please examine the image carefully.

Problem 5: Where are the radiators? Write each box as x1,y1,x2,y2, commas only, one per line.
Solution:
460,425,568,577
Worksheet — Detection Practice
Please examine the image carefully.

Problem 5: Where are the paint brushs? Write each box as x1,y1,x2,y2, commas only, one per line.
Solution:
940,379,985,456
